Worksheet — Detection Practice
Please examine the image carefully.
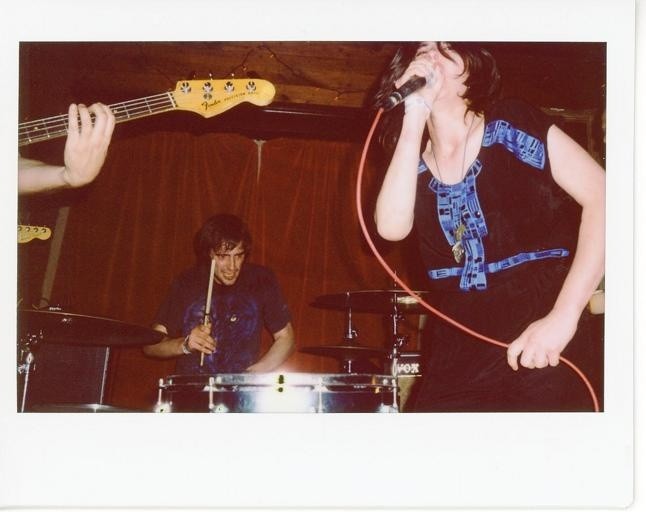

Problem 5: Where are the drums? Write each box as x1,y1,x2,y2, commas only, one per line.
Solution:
152,372,398,413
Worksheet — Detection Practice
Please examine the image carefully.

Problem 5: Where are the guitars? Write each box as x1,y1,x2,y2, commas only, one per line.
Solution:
18,70,276,150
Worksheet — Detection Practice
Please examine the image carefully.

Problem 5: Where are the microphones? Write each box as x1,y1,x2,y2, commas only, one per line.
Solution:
380,68,435,112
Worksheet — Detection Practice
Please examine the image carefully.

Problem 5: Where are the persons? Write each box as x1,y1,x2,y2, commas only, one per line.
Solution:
370,42,605,410
18,102,116,196
141,212,296,412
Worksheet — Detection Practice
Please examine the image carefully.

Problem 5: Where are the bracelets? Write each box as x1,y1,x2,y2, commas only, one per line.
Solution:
60,165,73,188
183,335,194,356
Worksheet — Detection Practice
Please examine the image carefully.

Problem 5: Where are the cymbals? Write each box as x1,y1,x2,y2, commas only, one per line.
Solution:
19,305,167,348
299,343,380,364
315,287,433,315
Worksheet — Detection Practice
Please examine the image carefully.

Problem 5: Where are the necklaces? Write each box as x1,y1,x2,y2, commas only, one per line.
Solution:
218,284,237,322
431,113,476,265
